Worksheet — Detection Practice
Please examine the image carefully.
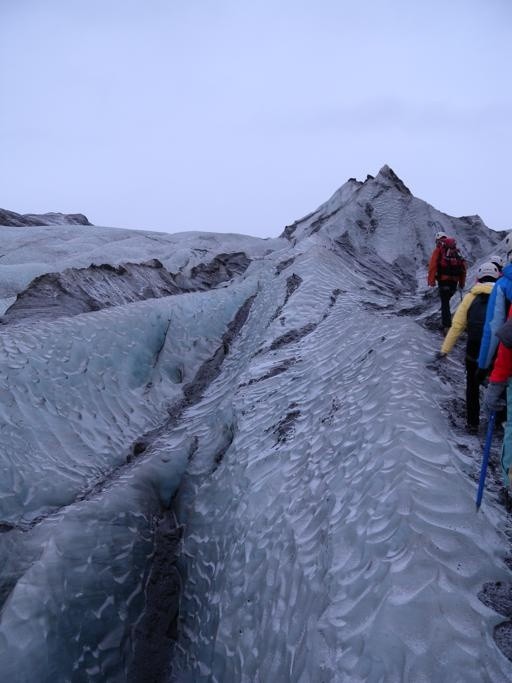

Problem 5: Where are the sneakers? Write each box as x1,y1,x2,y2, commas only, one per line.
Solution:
444,327,450,336
463,424,477,433
497,487,512,514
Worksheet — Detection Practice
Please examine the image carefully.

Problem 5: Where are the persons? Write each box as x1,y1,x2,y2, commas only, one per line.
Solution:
426,231,466,333
437,248,510,515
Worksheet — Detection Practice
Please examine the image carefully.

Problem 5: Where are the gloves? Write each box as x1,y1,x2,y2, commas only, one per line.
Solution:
432,350,446,362
473,367,487,383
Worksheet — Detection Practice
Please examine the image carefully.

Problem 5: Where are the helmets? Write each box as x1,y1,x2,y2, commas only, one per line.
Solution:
504,232,512,262
490,256,503,267
477,263,499,280
435,232,447,243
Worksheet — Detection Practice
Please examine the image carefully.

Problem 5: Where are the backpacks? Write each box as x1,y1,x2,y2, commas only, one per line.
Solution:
439,246,461,283
